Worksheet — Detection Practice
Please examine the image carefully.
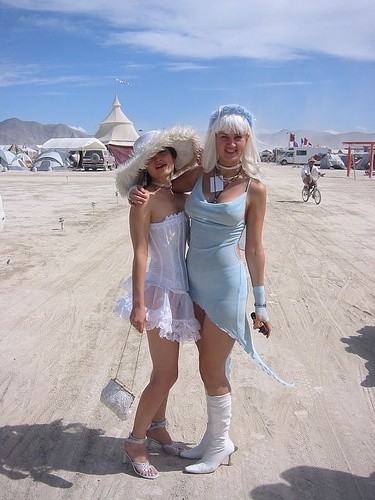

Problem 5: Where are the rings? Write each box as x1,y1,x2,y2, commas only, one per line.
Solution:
131,200,134,204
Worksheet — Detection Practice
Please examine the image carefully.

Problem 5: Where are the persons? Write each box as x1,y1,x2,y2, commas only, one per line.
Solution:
301,157,323,197
128,104,272,475
115,126,205,480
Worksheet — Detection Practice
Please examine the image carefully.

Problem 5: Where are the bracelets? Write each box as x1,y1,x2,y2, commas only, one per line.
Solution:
253,302,267,308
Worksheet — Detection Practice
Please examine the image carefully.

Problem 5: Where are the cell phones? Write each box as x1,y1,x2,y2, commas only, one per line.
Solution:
251,312,268,334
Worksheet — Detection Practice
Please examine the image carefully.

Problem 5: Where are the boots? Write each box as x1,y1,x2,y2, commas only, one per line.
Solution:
181,391,238,474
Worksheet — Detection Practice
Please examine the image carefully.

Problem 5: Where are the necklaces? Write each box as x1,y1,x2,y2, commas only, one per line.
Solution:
212,159,242,204
151,180,173,193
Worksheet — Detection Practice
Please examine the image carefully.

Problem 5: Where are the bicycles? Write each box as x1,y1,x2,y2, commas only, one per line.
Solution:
302,172,326,205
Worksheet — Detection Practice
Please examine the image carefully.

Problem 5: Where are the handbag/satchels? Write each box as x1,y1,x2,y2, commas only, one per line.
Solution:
100,378,136,421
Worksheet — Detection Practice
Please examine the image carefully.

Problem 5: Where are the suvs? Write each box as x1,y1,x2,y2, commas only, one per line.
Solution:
82,149,116,171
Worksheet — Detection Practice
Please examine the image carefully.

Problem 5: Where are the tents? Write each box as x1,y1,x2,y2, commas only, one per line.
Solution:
0,96,140,172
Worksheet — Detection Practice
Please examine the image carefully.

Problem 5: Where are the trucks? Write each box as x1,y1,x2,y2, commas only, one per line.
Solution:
278,147,332,165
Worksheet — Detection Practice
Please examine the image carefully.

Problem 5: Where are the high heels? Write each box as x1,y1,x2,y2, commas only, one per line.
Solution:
122,433,160,479
146,418,183,455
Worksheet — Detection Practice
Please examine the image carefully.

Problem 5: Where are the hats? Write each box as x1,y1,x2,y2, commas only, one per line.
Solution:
308,157,317,164
113,126,205,199
